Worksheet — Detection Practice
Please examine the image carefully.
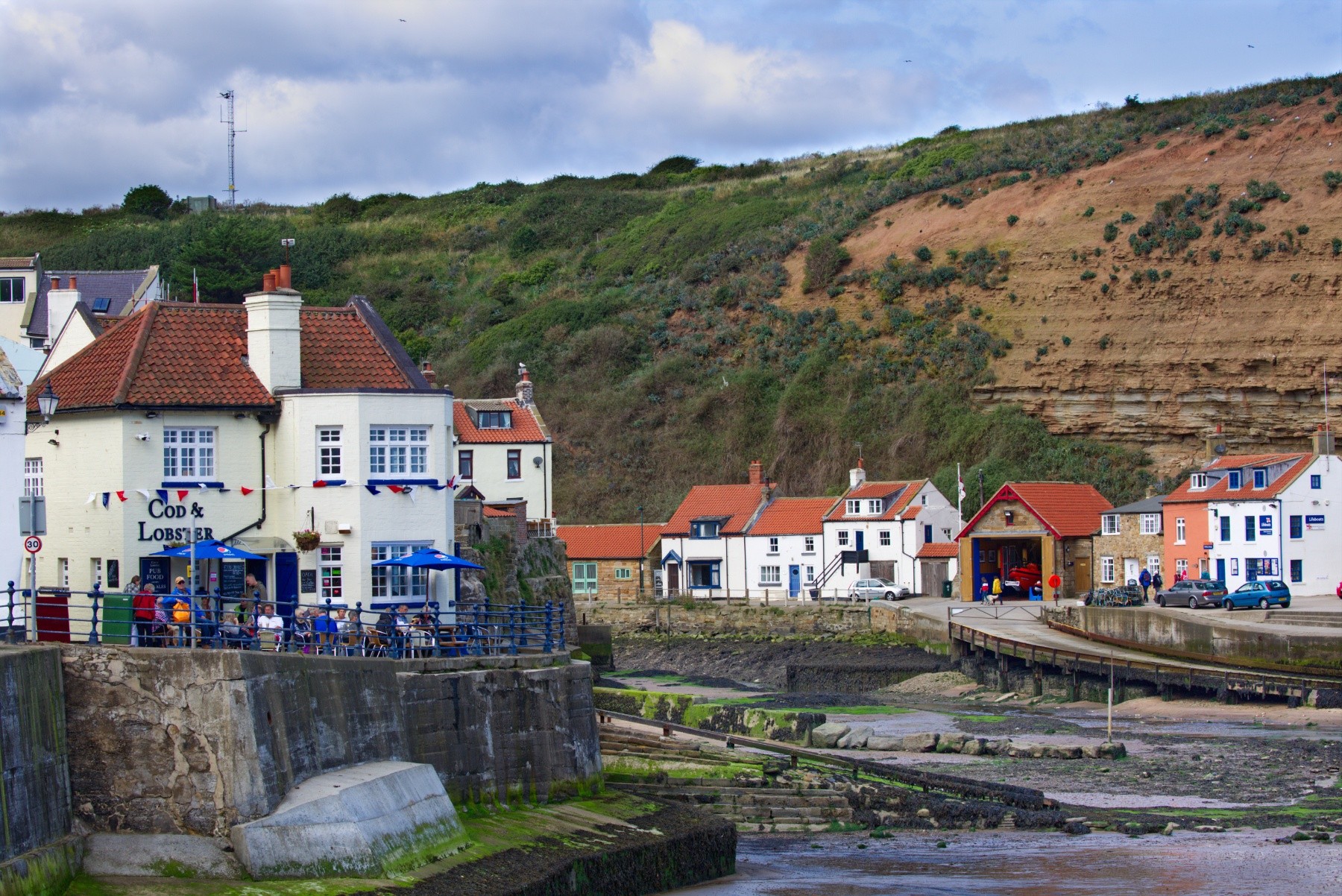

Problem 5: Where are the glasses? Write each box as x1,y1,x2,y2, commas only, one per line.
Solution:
402,608,407,611
300,613,304,615
178,581,185,584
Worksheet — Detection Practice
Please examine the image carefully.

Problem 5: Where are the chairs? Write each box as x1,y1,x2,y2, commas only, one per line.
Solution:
152,618,508,659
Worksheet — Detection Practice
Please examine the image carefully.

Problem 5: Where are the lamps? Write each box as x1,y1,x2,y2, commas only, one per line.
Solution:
1269,504,1276,508
26,378,59,433
145,412,157,418
402,486,413,494
338,523,352,535
235,414,246,419
47,440,59,446
1004,510,1014,517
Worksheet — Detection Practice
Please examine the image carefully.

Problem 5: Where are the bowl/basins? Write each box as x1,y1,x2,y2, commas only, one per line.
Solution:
409,629,416,631
398,624,409,630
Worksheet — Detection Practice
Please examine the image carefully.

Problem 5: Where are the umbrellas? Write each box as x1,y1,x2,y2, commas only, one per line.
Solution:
372,547,486,624
149,539,270,619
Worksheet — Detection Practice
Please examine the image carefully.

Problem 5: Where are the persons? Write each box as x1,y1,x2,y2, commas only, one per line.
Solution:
161,577,214,649
133,583,156,647
412,605,442,625
198,597,215,621
396,604,408,624
290,607,363,645
226,593,284,645
377,608,393,644
1139,566,1151,602
1153,571,1161,602
980,577,991,606
246,573,267,616
124,576,142,593
152,597,174,645
992,576,1003,605
1174,570,1187,585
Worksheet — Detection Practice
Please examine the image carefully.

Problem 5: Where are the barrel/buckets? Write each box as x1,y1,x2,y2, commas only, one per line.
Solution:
809,588,819,600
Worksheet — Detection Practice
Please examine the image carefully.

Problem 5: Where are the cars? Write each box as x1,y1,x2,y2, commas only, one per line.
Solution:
848,577,910,602
1153,579,1229,608
1220,579,1292,610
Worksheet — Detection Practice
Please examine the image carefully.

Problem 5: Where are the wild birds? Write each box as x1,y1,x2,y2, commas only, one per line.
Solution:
905,60,913,62
399,19,406,22
1247,45,1255,48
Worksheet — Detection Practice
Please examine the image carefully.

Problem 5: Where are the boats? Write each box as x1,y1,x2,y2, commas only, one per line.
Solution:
1007,563,1042,594
1034,580,1042,594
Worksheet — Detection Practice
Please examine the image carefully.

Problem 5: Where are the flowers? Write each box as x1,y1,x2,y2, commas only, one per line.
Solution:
293,528,320,553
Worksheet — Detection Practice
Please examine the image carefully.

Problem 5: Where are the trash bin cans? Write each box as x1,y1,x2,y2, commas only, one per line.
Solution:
941,580,953,598
102,594,133,645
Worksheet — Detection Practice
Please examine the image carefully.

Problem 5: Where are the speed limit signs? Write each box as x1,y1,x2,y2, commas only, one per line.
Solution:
25,536,42,553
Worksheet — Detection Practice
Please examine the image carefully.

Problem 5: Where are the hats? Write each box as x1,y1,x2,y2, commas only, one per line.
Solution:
318,608,326,614
175,576,185,584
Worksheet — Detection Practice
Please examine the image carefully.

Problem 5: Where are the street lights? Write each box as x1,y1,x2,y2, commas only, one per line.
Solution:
742,534,748,598
637,506,645,594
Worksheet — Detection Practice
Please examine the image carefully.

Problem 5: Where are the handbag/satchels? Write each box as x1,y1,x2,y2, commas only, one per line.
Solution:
173,602,190,623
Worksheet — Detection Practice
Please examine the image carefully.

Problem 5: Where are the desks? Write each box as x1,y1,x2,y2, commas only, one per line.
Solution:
416,625,461,660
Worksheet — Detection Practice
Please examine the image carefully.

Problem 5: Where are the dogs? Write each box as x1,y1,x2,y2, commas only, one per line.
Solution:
983,594,999,605
